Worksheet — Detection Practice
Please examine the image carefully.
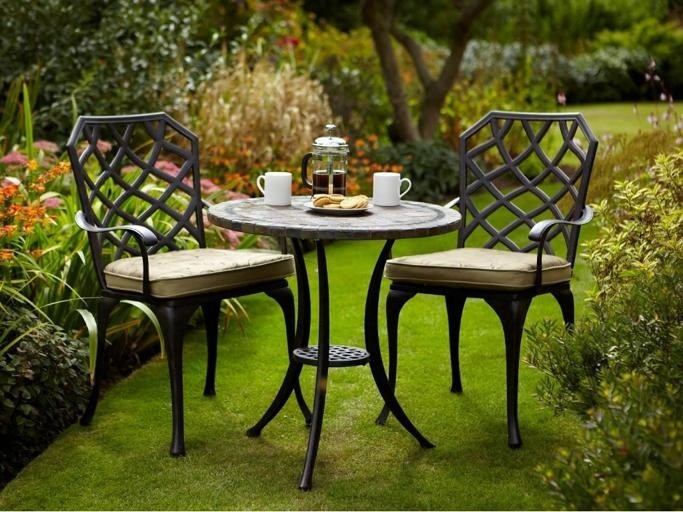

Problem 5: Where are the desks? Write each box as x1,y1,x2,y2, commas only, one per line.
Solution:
208,195,462,491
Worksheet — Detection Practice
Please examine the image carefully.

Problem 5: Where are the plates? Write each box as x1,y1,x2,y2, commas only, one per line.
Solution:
304,201,372,216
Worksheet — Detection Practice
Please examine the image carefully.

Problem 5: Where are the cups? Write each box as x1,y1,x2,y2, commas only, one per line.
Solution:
256,172,295,206
372,171,411,206
299,125,348,195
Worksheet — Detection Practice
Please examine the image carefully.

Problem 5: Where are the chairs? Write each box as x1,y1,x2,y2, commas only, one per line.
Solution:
67,112,314,457
375,110,599,448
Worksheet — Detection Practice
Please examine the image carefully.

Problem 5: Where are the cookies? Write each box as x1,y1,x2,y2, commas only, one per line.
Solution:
313,194,369,208
